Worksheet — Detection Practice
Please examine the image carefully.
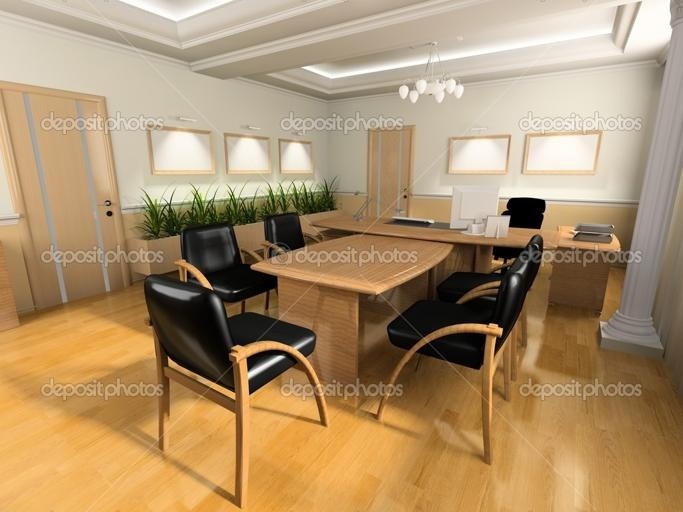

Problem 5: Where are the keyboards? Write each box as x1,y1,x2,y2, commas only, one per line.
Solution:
392,217,434,224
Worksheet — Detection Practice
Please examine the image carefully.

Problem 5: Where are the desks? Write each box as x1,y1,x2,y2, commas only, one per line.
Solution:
309,211,561,311
249,233,455,410
548,226,621,311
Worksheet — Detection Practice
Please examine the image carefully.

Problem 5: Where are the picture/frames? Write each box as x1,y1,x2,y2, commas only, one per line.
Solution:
521,129,603,175
448,134,511,175
146,124,217,177
224,132,274,176
278,138,315,175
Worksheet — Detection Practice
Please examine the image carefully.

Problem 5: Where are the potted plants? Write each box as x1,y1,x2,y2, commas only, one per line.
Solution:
128,174,343,277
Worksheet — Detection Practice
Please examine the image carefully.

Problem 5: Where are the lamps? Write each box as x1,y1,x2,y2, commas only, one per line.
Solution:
398,41,464,104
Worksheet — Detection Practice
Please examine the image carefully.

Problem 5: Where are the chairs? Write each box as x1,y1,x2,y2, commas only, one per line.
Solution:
374,251,534,466
492,197,545,266
261,212,321,258
173,220,277,313
144,273,330,509
436,233,544,381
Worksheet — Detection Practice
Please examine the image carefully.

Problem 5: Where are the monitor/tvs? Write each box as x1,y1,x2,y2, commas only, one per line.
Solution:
450,183,500,236
